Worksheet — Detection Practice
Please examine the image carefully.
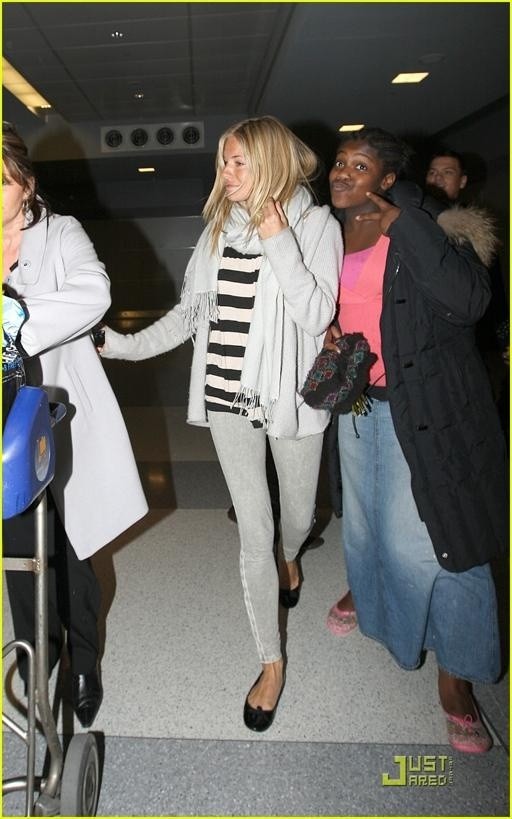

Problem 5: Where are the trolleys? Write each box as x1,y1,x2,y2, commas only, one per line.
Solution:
0,380,102,817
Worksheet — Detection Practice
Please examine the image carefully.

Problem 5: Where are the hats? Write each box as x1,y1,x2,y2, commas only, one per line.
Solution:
300,332,378,416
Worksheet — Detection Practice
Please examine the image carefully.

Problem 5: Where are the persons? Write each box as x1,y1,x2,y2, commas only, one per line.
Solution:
323,128,511,752
3,121,150,729
421,151,468,200
95,115,344,733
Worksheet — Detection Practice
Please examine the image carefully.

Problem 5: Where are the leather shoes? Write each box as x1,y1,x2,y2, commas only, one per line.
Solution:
71,661,103,728
244,554,493,754
